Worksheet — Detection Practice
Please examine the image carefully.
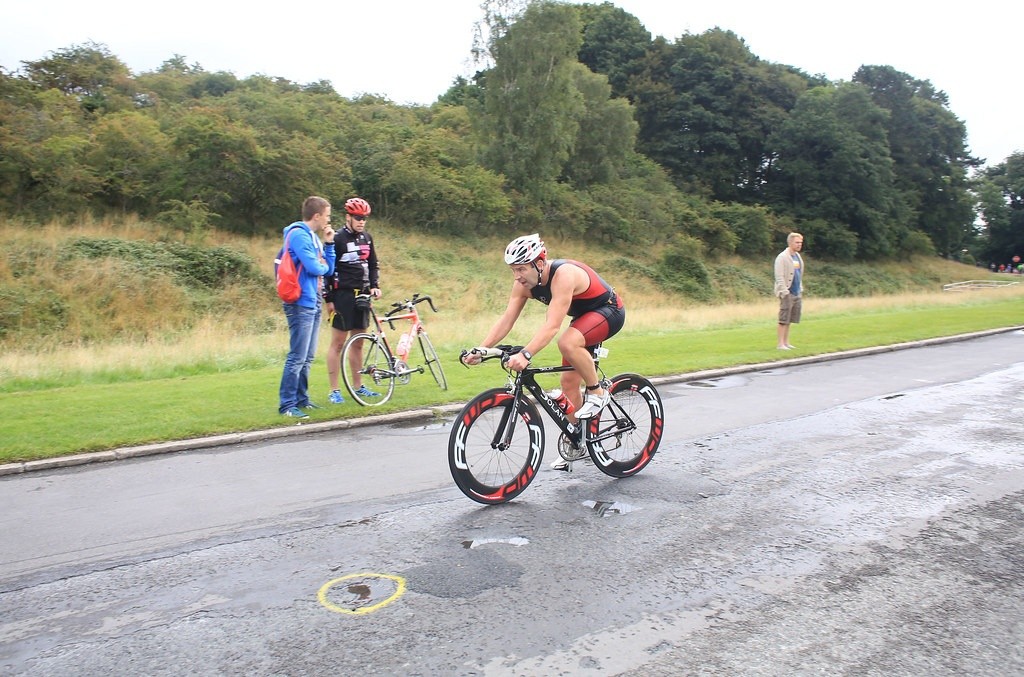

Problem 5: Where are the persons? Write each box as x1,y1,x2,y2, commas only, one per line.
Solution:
321,198,382,404
959,248,1024,274
774,232,804,350
463,234,625,470
274,196,336,420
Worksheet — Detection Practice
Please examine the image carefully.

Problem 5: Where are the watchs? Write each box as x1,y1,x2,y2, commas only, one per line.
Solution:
519,349,531,361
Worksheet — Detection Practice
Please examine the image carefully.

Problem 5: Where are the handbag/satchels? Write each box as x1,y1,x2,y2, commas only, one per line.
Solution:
277,227,303,303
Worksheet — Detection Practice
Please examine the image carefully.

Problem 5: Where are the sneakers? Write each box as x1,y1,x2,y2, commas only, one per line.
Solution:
551,452,573,472
328,389,345,405
354,384,381,397
281,407,309,419
575,389,611,418
305,403,323,411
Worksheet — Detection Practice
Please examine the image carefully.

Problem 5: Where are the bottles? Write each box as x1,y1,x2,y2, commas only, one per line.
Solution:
550,389,575,415
396,332,408,355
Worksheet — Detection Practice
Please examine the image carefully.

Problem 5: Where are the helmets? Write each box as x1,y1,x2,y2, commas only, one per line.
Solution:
344,198,371,215
504,234,547,265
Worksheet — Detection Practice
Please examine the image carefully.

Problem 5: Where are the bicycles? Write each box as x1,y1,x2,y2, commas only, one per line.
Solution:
449,346,667,506
339,293,449,407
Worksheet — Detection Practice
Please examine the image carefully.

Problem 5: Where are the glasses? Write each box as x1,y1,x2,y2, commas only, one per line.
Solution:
350,214,366,221
